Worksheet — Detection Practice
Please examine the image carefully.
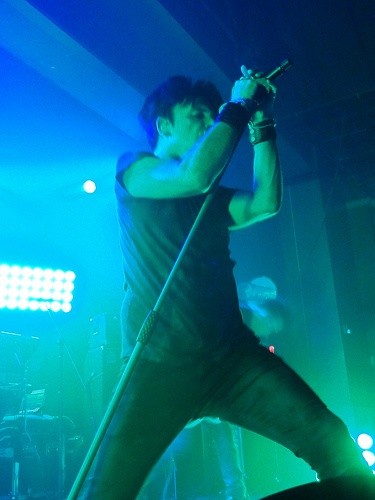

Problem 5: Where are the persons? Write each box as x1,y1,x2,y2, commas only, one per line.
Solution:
162,293,288,500
79,64,371,499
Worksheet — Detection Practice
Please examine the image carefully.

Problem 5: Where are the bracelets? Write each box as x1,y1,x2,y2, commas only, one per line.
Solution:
215,102,249,133
247,119,277,145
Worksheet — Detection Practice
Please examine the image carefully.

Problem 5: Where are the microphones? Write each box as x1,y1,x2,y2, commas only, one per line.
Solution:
219,57,294,115
